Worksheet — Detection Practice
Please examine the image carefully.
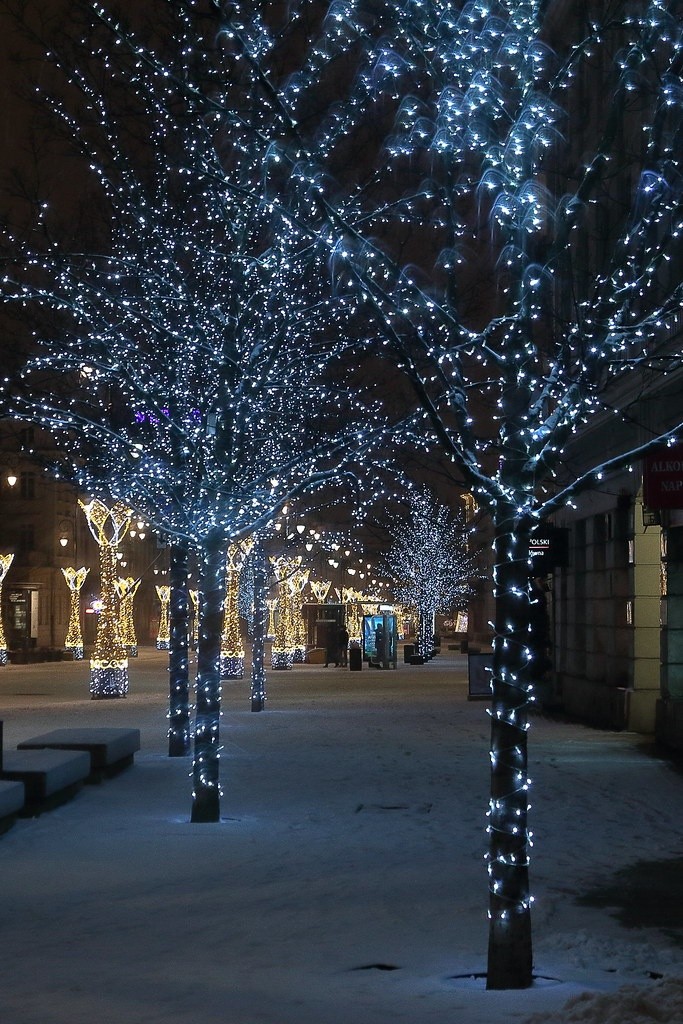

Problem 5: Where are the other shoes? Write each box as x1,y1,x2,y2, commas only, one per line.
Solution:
339,662,347,667
323,662,339,668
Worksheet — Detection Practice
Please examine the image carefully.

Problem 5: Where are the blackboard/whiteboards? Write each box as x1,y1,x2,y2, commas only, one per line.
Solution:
468,652,494,694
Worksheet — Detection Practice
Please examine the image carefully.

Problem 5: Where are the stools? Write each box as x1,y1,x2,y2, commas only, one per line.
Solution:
17,727,140,779
0,780,25,834
2,750,91,810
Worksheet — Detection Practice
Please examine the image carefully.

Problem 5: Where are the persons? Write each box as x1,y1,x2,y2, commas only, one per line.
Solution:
337,624,349,668
322,625,340,668
374,622,384,667
